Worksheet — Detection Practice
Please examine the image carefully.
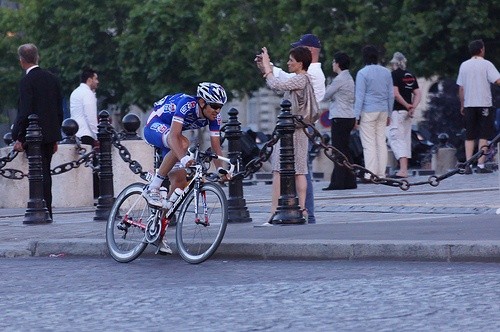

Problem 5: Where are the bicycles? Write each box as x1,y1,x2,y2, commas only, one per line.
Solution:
105,143,240,265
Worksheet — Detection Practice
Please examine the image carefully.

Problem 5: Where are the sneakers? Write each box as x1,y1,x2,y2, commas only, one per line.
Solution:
142,185,163,207
152,239,172,254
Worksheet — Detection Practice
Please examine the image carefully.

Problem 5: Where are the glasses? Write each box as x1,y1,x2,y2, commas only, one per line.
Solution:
210,103,222,110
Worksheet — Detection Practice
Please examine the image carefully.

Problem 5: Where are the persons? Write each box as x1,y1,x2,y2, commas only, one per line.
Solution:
143,82,232,256
321,53,357,190
69,67,99,146
456,39,500,174
386,52,422,178
430,133,456,171
269,34,326,223
255,46,313,227
10,44,64,220
353,44,394,185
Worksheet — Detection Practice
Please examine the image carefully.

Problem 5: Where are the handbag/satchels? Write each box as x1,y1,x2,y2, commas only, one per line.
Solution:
288,76,320,127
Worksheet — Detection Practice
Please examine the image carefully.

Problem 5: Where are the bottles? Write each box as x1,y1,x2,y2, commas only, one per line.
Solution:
160,186,167,200
166,185,183,205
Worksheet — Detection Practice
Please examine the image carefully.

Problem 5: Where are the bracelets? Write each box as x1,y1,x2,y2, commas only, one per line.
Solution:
263,70,272,78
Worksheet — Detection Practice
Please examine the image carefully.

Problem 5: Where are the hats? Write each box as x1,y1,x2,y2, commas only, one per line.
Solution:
290,34,321,48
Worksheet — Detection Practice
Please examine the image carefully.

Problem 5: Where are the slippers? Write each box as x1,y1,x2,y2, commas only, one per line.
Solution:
389,171,405,178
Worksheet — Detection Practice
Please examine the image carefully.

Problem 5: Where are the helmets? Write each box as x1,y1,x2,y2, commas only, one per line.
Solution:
197,81,227,104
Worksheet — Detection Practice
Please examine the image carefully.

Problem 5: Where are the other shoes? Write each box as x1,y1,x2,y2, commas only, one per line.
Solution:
322,186,333,190
476,166,492,173
466,168,473,174
